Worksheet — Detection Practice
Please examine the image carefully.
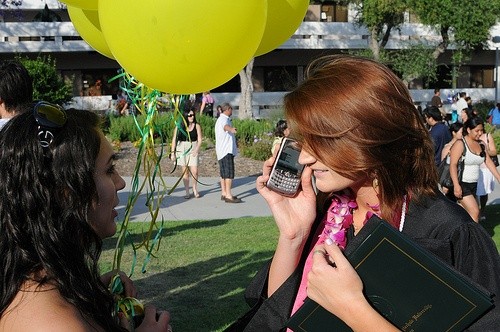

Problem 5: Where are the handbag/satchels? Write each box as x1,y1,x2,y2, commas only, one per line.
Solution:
486,133,499,167
203,97,213,113
437,139,467,189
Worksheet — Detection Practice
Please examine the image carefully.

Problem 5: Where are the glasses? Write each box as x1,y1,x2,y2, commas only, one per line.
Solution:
231,107,232,110
34,100,67,171
424,117,427,119
188,113,194,117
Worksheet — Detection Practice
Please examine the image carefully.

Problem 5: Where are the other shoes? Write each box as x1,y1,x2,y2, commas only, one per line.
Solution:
193,192,201,198
221,195,237,200
225,198,241,203
185,195,191,199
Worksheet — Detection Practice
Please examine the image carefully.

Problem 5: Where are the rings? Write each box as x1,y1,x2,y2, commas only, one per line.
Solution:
312,250,327,258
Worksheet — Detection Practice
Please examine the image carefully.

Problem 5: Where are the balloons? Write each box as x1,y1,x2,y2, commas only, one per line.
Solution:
57,0,311,94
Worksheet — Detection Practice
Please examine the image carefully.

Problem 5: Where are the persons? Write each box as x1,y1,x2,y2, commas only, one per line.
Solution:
245,55,500,332
215,103,242,203
431,88,447,123
217,105,223,118
170,106,202,200
423,85,500,225
89,79,102,96
116,98,130,116
488,103,500,129
270,120,291,158
0,109,173,332
103,82,114,95
0,60,33,132
199,92,214,118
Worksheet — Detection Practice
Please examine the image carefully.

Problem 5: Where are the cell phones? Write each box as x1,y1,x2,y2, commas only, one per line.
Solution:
265,137,307,197
